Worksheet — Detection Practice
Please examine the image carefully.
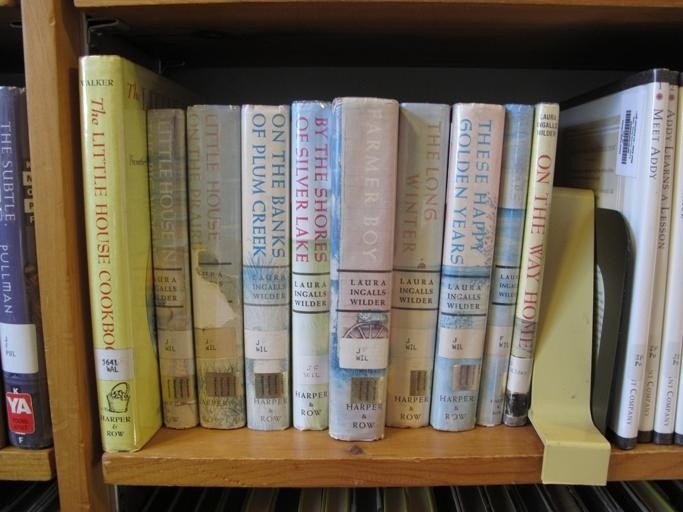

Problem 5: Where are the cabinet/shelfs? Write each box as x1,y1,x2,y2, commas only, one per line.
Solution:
1,0,683,512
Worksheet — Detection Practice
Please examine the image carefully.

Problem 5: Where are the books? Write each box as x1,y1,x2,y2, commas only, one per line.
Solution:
0,84,53,451
118,479,683,512
76,55,682,452
